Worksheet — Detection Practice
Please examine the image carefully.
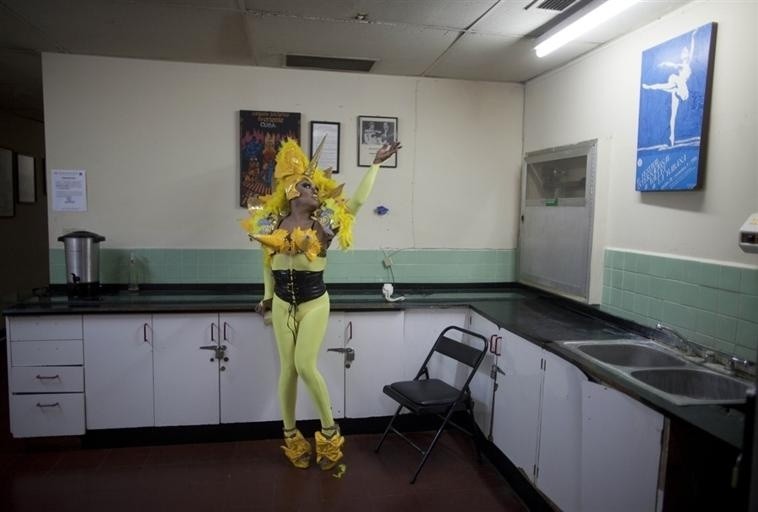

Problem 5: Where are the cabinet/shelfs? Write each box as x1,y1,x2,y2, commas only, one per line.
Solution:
458,309,541,489
5,309,84,439
405,302,470,418
296,308,404,419
535,351,668,512
83,310,154,431
153,309,281,426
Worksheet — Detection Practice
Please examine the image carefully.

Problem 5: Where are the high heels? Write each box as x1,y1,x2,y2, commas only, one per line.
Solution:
280,427,314,468
315,423,345,471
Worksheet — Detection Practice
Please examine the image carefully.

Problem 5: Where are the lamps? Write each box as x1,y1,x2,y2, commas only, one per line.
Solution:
532,0,642,58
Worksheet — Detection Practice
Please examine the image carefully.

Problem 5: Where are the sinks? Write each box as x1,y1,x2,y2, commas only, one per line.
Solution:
552,339,692,367
605,367,758,406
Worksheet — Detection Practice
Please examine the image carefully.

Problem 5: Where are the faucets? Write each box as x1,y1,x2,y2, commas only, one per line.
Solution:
656,323,709,363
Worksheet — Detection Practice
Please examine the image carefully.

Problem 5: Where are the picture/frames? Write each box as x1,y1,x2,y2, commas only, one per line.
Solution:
17,152,38,205
0,147,18,220
309,120,340,177
356,115,399,169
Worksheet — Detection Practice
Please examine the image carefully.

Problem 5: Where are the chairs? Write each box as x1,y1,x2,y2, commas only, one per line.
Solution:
375,324,488,483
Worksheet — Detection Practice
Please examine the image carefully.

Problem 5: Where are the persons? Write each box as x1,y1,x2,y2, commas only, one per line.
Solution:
363,120,380,145
377,123,395,145
640,24,703,151
235,133,404,473
